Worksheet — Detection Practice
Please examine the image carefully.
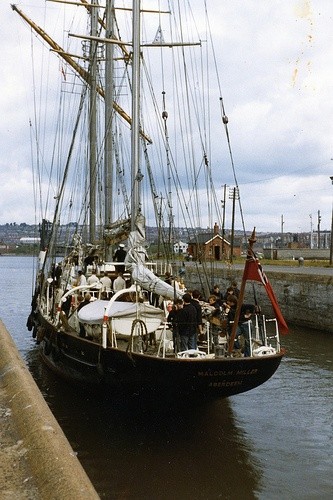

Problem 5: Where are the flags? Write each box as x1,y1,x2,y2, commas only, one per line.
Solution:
243,247,288,336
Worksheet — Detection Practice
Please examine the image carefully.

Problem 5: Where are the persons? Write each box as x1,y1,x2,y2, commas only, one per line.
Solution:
73,251,79,265
59,289,72,332
77,293,91,338
113,244,127,273
143,272,256,358
84,255,99,275
198,250,204,264
51,263,63,287
78,269,126,293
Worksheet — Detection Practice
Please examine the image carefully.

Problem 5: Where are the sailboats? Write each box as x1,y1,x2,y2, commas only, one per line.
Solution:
26,1,287,408
